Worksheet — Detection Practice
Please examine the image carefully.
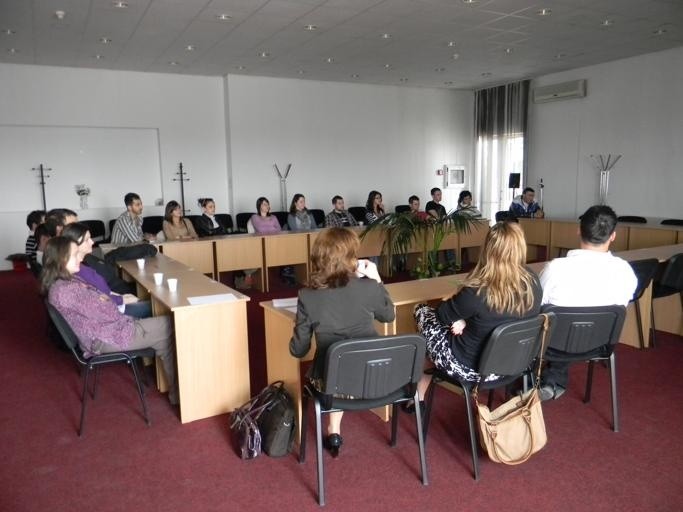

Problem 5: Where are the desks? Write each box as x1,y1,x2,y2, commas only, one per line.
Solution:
378,276,473,421
524,262,553,280
551,220,629,258
153,277,252,424
459,219,492,267
613,248,656,349
678,225,683,244
390,224,460,275
628,224,678,250
122,261,193,362
628,224,655,251
310,227,390,280
256,297,391,455
161,236,215,282
263,232,310,293
102,246,174,284
516,217,551,264
211,235,264,293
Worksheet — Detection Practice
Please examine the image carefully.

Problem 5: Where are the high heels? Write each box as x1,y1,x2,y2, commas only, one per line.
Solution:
401,400,425,414
328,433,343,457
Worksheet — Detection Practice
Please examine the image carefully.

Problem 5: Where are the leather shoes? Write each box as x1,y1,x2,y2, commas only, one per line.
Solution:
538,385,554,402
552,384,565,399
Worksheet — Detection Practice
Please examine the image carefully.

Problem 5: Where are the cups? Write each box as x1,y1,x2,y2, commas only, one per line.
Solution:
136,259,144,270
153,273,163,286
166,279,177,292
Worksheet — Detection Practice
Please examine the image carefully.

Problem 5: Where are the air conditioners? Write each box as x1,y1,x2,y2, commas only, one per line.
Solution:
533,79,586,104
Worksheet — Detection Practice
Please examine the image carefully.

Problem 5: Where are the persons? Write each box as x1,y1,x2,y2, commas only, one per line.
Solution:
195,198,228,236
40,237,180,407
537,206,638,400
403,219,543,417
288,194,317,230
457,190,483,218
58,223,153,317
251,197,282,233
289,227,395,457
162,201,198,241
110,193,156,244
25,210,47,255
425,188,446,220
34,222,53,263
404,195,435,222
366,190,394,226
510,188,546,218
43,208,79,237
325,195,361,230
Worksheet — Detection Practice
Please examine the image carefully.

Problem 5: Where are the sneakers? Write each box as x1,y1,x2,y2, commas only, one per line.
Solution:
169,391,180,405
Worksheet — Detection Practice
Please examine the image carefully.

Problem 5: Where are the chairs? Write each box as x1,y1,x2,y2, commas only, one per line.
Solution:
652,252,683,348
539,303,627,434
215,214,235,232
395,205,412,215
615,215,648,224
626,258,659,349
348,206,366,223
270,211,289,228
494,211,510,222
660,219,683,226
237,212,254,231
80,220,105,244
421,311,560,481
109,219,116,236
184,216,205,234
141,216,164,234
28,257,47,287
307,208,324,227
298,333,428,506
43,297,154,439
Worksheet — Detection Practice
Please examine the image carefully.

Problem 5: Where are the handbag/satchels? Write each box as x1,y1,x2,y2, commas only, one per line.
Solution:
229,389,276,460
253,381,297,458
472,314,547,465
